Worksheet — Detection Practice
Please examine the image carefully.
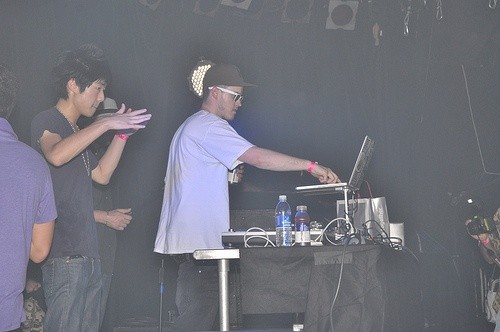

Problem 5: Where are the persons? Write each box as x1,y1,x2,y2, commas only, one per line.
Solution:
154,63,344,332
0,47,153,332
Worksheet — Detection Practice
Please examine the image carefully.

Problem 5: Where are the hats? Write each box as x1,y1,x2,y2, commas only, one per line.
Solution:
203,63,257,88
92,97,119,119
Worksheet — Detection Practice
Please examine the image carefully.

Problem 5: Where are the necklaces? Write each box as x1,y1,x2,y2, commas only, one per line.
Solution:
62,113,90,178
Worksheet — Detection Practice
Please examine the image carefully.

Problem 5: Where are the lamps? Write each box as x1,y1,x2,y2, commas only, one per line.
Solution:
139,0,359,30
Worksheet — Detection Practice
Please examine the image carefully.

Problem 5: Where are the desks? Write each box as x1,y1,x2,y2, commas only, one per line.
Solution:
192,244,426,332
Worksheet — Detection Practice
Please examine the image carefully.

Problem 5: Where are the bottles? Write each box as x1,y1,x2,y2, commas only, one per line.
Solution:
294,206,310,246
274,195,292,247
228,165,239,185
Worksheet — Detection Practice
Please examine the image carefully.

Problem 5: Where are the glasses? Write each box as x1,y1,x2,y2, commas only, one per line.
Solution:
208,86,245,102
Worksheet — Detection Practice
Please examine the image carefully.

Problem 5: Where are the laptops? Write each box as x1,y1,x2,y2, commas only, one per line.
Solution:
296,135,376,190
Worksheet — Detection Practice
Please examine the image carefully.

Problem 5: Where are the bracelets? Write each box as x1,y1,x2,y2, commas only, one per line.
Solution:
104,211,110,227
307,161,317,173
114,131,129,141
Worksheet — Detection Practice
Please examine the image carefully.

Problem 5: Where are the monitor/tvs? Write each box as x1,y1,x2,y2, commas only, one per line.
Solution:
336,197,369,235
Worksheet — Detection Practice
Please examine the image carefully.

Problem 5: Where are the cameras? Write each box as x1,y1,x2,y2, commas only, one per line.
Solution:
467,217,494,235
